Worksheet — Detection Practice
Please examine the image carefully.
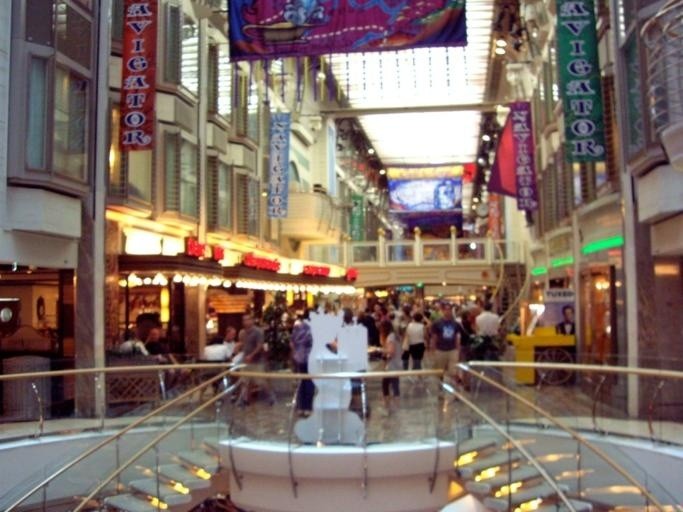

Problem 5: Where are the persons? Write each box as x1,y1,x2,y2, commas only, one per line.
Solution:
554,302,576,336
118,287,503,418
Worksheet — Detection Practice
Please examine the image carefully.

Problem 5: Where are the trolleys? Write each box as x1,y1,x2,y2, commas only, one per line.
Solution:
505,323,575,387
